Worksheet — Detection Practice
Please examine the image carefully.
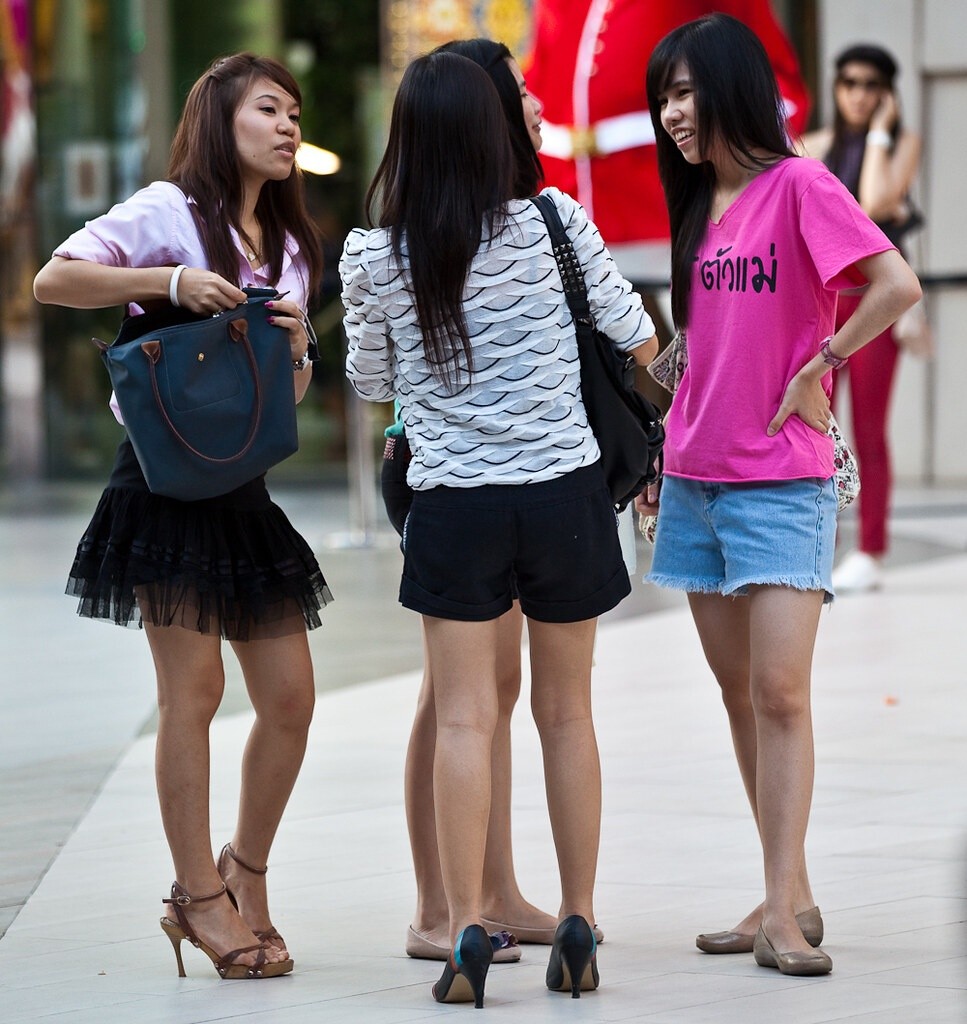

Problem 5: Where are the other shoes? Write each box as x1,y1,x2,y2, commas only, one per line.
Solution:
829,549,885,592
478,916,604,946
696,905,823,954
753,923,833,976
405,923,522,964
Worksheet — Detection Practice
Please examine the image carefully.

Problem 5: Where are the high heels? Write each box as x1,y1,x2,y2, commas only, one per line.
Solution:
545,915,600,998
159,881,294,980
216,842,287,951
432,924,494,1009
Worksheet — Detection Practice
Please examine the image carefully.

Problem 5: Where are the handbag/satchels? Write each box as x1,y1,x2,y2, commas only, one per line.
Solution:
639,325,862,547
91,262,299,501
529,194,666,513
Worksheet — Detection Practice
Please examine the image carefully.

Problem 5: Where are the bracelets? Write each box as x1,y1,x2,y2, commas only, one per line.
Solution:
170,265,187,306
862,130,891,150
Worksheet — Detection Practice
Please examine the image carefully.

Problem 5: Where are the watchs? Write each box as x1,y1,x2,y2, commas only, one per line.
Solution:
290,351,309,371
819,335,848,371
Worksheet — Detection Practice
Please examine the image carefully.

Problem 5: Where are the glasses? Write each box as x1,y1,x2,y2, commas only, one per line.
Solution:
839,75,882,92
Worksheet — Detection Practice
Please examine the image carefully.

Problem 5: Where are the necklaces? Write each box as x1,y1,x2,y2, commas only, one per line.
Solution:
245,212,262,262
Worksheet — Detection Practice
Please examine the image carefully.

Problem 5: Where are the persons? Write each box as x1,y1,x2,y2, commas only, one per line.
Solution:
378,40,604,962
640,13,924,980
34,52,332,975
791,45,919,592
336,52,657,1009
